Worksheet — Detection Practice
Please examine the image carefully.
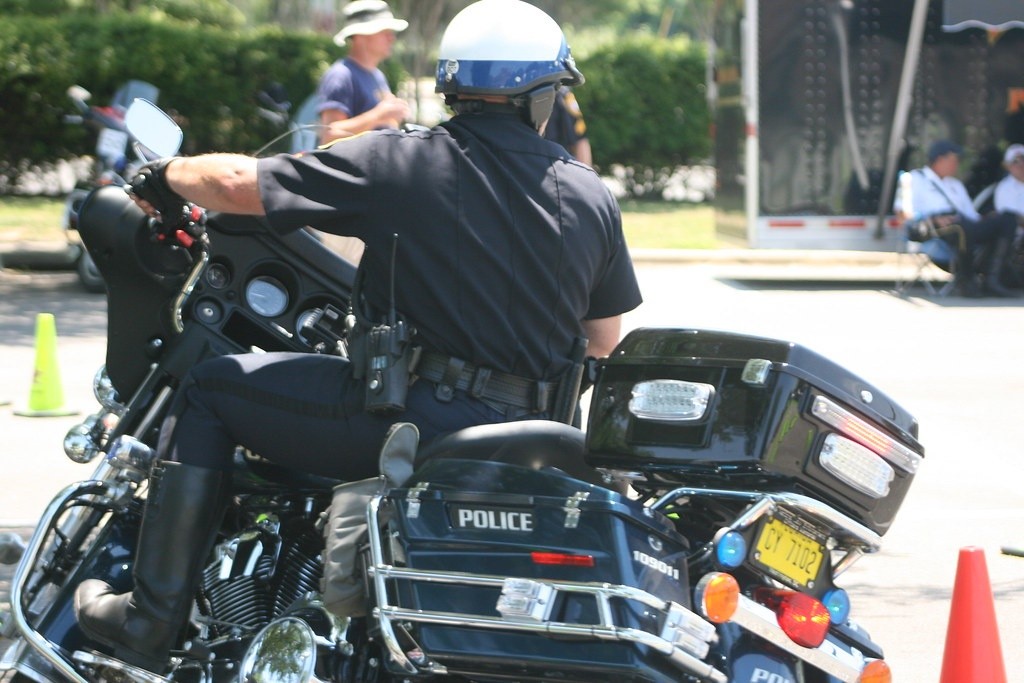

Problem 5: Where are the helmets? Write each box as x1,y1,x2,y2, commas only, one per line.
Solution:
433,1,584,102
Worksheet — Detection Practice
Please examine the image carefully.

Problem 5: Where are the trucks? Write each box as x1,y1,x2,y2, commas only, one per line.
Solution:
715,0,1024,290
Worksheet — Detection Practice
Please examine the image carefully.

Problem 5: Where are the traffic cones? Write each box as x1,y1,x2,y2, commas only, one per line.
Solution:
14,312,80,417
939,546,1006,683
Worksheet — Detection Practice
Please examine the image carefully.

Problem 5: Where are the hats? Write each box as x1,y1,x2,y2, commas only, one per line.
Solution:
928,140,962,161
331,1,413,45
1002,144,1022,161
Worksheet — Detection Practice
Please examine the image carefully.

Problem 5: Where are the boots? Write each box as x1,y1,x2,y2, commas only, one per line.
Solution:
984,241,1016,297
71,457,234,672
956,253,981,295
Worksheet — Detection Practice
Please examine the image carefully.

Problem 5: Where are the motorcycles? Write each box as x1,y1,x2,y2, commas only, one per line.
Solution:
0,97,925,683
67,79,160,294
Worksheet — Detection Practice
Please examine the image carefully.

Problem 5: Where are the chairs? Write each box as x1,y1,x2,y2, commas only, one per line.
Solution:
894,166,968,296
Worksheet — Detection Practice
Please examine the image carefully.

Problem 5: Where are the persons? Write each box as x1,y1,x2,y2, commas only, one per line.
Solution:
542,88,591,166
72,0,643,671
902,138,1016,297
993,144,1024,239
317,0,410,148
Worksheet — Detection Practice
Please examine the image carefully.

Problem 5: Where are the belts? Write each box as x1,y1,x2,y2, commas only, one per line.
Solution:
411,341,564,416
